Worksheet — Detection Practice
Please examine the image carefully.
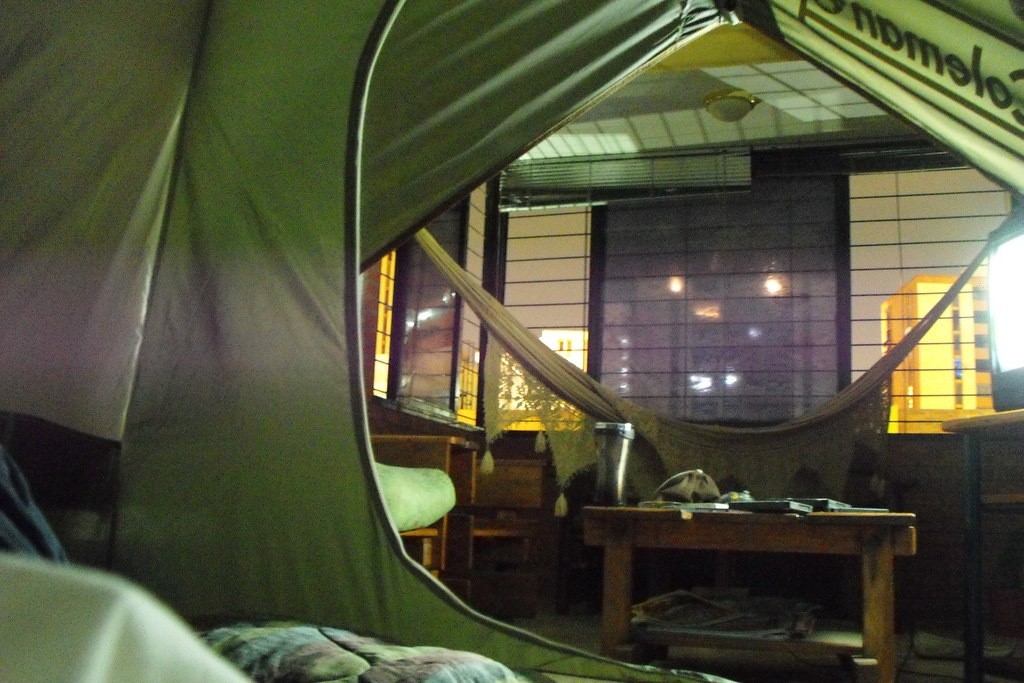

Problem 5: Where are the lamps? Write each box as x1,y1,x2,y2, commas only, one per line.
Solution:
702,89,756,122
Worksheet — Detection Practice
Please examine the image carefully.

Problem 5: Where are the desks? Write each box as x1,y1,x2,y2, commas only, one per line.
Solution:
581,506,917,683
941,409,1024,683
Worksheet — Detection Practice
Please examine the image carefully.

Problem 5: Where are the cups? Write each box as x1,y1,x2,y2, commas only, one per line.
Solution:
593,422,635,505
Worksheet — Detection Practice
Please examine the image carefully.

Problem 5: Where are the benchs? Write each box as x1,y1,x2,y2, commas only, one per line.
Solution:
372,435,549,619
